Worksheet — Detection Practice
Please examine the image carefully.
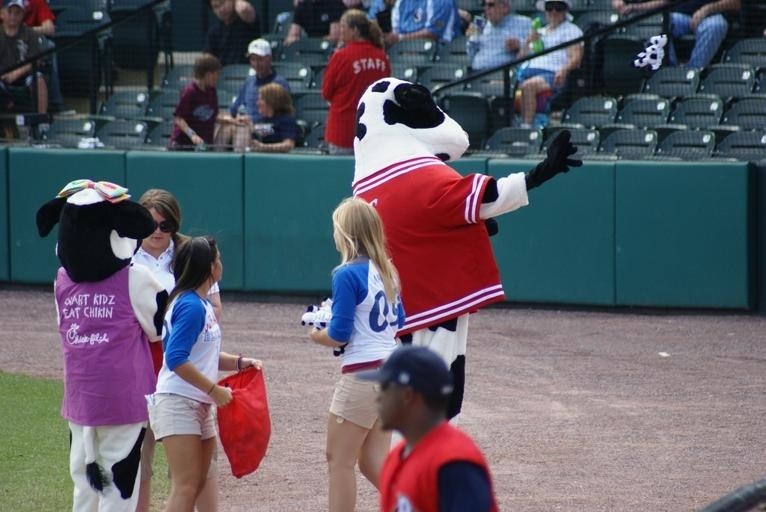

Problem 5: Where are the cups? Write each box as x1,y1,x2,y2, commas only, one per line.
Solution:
469,15,485,46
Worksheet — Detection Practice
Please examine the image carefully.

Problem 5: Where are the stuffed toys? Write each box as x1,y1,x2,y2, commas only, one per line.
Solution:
32,176,169,512
348,75,588,454
299,294,345,357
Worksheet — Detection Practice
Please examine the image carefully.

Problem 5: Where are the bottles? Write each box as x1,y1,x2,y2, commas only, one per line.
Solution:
528,18,543,53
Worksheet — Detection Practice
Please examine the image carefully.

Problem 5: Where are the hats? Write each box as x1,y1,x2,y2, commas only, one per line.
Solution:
0,0,24,9
356,346,455,397
246,37,273,57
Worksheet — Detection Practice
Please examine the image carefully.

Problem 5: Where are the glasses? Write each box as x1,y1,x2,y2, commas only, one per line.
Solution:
154,220,175,232
546,5,564,11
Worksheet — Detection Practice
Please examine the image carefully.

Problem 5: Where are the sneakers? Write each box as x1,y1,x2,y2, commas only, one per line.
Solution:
28,116,52,144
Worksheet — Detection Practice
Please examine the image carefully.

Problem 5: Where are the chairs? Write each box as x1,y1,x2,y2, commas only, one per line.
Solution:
0,0,766,168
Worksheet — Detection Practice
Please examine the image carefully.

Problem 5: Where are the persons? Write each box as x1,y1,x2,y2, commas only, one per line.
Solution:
283,1,487,47
129,184,223,512
203,1,257,67
212,38,292,144
142,235,265,512
612,1,669,18
212,82,298,152
166,53,251,154
303,196,407,512
661,3,766,75
321,9,391,154
466,1,584,146
0,0,76,145
355,341,502,511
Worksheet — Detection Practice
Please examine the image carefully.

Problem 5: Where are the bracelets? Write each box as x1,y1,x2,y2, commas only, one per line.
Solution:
207,381,218,395
236,356,243,370
